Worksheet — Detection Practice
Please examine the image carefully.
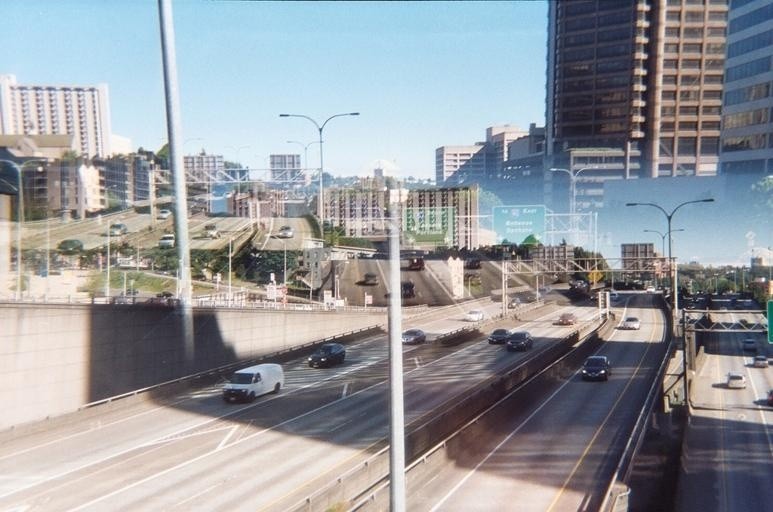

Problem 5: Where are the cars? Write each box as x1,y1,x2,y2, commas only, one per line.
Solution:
464,309,483,323
56,209,219,255
278,224,292,239
582,354,611,381
488,327,534,353
559,313,577,325
114,289,177,306
623,316,641,331
361,252,426,299
463,259,485,288
307,343,346,368
400,329,426,345
727,299,773,406
504,277,619,309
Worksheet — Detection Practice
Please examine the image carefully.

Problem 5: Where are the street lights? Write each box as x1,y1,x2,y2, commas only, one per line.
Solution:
549,165,599,238
626,199,712,296
287,138,323,168
278,110,358,224
0,157,51,299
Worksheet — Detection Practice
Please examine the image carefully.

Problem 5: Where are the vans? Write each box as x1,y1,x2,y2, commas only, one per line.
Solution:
222,363,285,403
